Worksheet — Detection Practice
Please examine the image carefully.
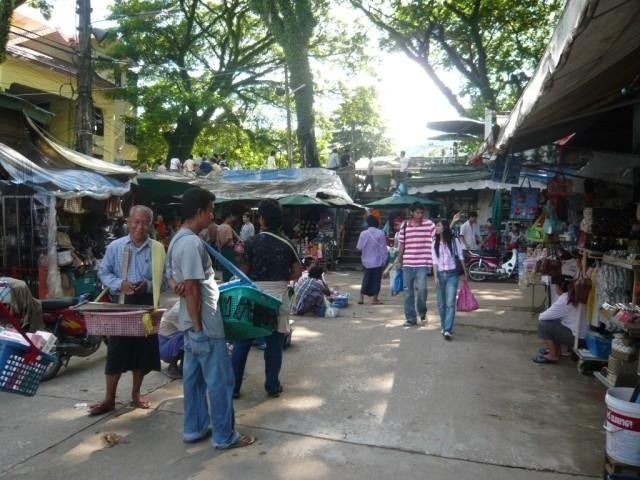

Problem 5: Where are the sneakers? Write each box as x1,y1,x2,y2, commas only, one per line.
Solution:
441,329,453,341
267,384,283,398
404,319,416,326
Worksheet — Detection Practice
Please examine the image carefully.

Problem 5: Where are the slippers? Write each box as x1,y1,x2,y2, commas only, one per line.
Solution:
130,398,152,408
532,355,554,364
185,428,213,441
211,432,255,447
539,348,551,354
88,400,118,414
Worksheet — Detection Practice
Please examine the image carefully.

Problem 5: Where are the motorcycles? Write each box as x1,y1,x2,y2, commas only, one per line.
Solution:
0,279,99,382
463,241,519,282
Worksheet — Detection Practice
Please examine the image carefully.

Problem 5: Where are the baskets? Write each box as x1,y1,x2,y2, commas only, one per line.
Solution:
77,302,170,339
233,240,245,257
216,285,284,342
0,338,56,398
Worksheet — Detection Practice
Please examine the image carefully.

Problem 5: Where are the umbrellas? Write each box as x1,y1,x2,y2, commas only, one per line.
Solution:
426,132,488,145
427,116,499,134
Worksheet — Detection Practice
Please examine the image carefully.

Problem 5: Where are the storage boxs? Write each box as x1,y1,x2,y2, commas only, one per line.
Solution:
584,334,611,358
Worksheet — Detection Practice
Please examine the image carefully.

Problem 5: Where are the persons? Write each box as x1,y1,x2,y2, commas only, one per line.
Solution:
157,298,185,379
124,211,220,247
531,275,588,365
140,158,149,173
165,187,255,451
459,212,487,277
367,207,383,230
327,148,339,170
478,217,497,251
394,201,463,327
288,265,330,317
88,204,166,415
237,210,256,278
505,223,522,251
431,218,469,341
341,147,350,167
357,214,389,306
449,208,460,236
391,150,410,195
267,148,277,169
157,148,233,180
293,257,316,294
215,210,238,283
229,197,301,399
395,217,403,256
360,154,375,194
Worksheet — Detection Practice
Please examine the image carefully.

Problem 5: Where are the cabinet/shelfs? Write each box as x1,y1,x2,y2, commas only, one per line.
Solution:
0,195,75,271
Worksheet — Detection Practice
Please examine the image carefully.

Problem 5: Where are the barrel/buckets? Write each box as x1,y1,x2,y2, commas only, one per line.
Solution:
603,387,640,469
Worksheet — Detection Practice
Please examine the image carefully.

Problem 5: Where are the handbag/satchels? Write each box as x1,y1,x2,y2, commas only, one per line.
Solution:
388,268,404,297
454,253,464,276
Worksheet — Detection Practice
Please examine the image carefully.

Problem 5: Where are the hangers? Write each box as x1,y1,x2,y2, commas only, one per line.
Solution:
591,260,627,282
